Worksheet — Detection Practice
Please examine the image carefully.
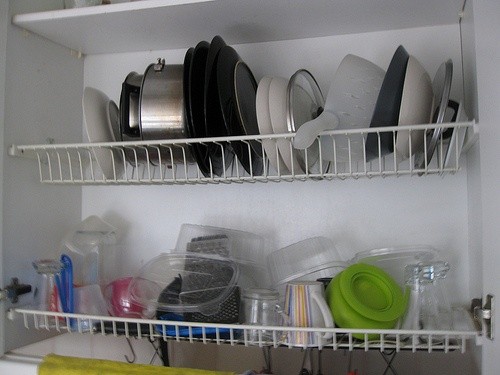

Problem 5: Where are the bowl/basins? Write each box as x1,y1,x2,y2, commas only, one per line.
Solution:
365,44,435,164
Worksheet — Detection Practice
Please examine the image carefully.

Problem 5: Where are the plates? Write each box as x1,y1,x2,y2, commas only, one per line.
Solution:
183,34,268,178
81,84,126,179
255,74,319,175
444,99,470,170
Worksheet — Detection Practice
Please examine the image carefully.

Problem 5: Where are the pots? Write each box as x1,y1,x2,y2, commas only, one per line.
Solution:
120,58,196,168
293,53,386,163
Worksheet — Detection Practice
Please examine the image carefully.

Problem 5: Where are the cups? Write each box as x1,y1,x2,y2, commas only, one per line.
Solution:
74,284,109,327
403,262,469,345
237,280,335,348
36,260,64,326
75,230,116,283
104,277,144,319
